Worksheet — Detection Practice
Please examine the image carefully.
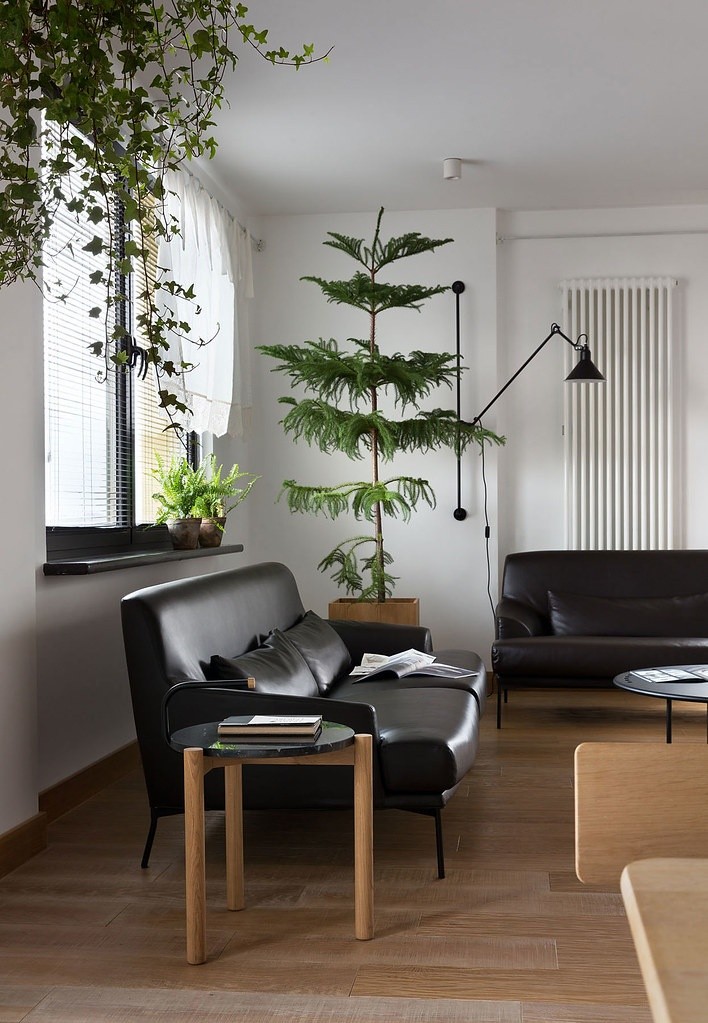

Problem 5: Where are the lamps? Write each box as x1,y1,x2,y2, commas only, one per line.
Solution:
443,158,461,179
451,280,606,521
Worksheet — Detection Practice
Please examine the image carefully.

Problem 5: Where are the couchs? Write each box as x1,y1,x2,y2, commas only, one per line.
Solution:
491,550,708,730
120,561,487,878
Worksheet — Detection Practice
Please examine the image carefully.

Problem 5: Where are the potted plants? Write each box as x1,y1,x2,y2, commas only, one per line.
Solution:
142,447,216,550
253,205,508,628
198,451,264,547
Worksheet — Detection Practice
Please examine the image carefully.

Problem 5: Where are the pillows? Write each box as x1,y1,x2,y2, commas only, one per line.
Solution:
209,628,321,696
548,588,708,636
269,610,354,697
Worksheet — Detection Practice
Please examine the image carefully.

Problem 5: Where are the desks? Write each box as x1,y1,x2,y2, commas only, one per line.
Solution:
619,858,708,1023
613,665,708,743
171,715,374,965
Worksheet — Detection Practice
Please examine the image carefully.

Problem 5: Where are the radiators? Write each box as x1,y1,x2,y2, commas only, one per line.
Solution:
557,276,678,550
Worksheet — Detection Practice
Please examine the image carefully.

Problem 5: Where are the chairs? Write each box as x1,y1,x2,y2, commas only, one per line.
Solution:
574,742,708,885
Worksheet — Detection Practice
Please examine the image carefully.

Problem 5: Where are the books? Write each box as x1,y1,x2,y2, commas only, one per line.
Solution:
629,667,708,682
350,649,479,685
219,727,322,744
218,715,322,734
